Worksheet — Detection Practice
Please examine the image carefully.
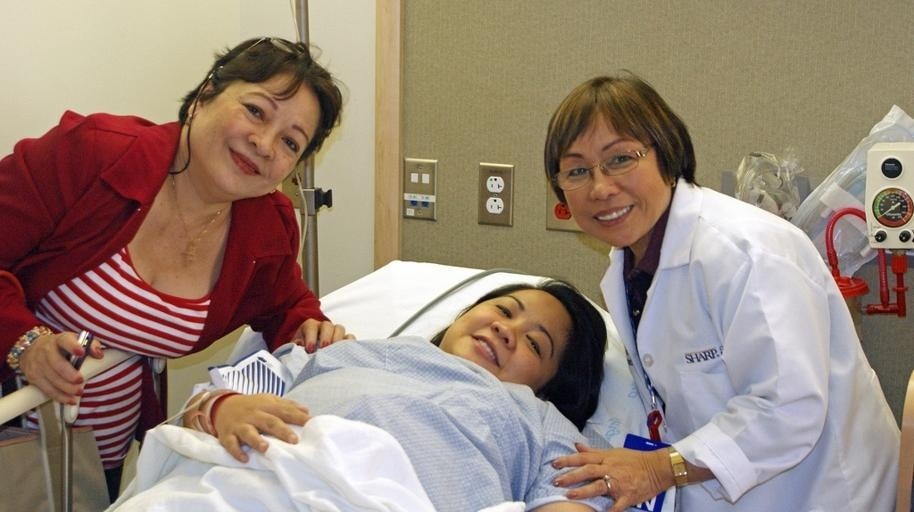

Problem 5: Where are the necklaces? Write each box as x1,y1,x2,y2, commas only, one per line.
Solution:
165,165,224,269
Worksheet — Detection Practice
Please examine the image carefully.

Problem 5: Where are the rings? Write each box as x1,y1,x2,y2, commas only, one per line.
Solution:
601,476,612,494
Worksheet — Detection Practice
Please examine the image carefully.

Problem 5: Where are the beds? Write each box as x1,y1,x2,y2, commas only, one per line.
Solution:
95,255,682,512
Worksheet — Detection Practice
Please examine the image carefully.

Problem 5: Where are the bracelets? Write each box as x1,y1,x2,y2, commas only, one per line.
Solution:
206,388,243,440
668,442,689,491
6,323,54,382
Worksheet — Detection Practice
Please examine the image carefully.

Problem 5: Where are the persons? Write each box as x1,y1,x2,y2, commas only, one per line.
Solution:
0,32,360,511
99,275,615,511
542,69,900,511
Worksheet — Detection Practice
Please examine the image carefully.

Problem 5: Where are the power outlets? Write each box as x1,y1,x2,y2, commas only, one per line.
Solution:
479,161,515,227
545,169,583,231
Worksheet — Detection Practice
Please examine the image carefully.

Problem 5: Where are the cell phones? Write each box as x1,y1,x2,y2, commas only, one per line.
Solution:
68,329,93,370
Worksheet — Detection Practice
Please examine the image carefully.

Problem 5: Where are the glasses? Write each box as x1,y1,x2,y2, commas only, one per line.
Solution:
553,146,651,192
216,32,314,70
